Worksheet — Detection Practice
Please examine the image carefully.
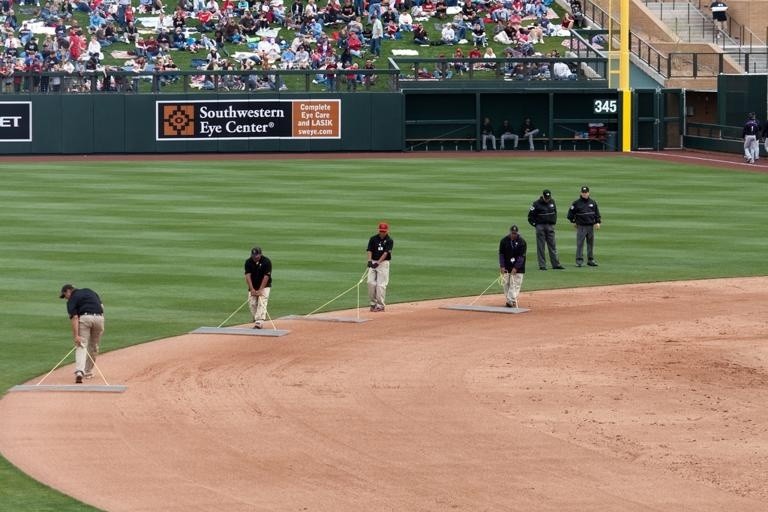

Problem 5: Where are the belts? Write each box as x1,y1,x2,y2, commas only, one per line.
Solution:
82,312,101,315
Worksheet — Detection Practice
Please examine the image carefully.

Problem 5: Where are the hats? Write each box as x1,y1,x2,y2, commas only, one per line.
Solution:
60,285,72,298
380,224,388,232
512,225,518,231
582,187,588,192
252,247,261,254
544,190,551,199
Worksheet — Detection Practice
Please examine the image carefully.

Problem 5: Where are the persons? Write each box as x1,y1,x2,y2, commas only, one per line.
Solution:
528,189,567,271
742,113,758,163
520,116,540,152
244,247,272,329
565,186,602,268
499,119,520,150
366,222,395,313
761,120,768,154
480,116,498,151
752,118,760,160
59,284,105,384
709,0,728,40
497,225,527,307
1,0,592,93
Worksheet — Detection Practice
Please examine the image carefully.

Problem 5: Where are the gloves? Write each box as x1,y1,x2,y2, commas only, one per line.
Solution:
372,263,378,268
368,261,372,268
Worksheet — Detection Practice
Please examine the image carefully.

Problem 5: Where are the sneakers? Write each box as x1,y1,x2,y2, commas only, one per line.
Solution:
76,372,82,383
744,156,759,164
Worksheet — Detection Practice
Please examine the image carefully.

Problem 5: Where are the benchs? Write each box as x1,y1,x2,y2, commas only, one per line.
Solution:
405,135,608,151
